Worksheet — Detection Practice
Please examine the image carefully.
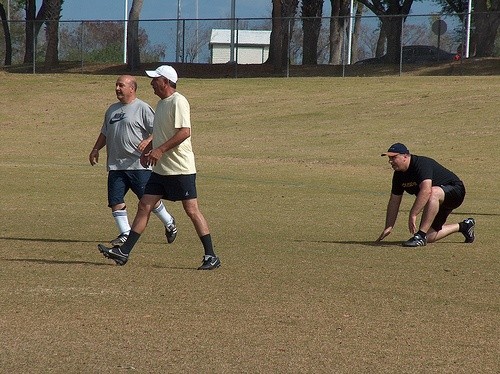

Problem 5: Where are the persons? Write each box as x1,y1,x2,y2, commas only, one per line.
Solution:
89,74,178,244
97,66,221,272
374,142,475,247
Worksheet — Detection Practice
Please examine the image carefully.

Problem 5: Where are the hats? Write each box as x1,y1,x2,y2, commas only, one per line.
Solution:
145,65,178,84
381,143,407,157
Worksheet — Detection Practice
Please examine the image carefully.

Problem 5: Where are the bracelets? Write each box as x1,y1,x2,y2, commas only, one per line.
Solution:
92,149,98,151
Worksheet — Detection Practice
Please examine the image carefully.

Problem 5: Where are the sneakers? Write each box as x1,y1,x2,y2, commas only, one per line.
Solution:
199,255,221,270
463,217,475,243
97,244,129,266
110,233,129,244
165,216,178,243
403,232,427,246
